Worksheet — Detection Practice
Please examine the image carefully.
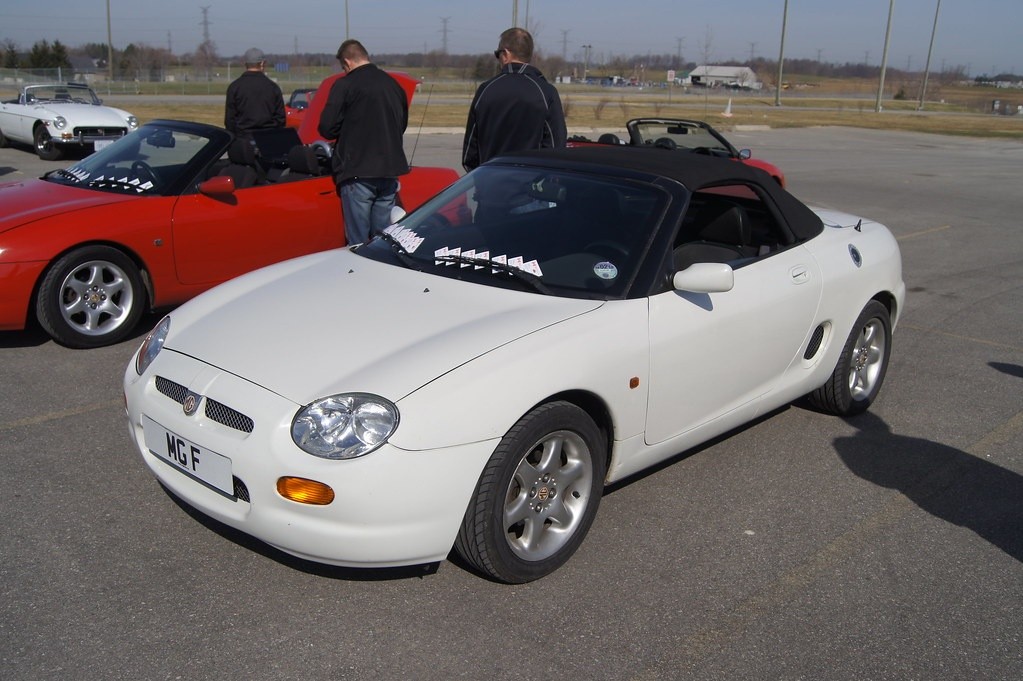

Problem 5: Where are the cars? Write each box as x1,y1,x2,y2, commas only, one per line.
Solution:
0,82,141,159
0,120,472,350
567,117,786,200
120,143,906,585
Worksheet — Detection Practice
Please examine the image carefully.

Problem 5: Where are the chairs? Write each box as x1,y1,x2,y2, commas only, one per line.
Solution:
566,186,629,254
275,144,319,183
216,136,258,191
669,198,753,276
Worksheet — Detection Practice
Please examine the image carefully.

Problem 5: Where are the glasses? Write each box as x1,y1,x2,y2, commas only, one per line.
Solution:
494,49,512,59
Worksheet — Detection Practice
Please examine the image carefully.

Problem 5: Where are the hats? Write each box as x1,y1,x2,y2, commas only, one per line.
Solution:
244,48,266,64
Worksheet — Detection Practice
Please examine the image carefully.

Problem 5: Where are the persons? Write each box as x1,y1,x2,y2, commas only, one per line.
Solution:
462,27,567,223
318,41,411,247
225,49,287,160
